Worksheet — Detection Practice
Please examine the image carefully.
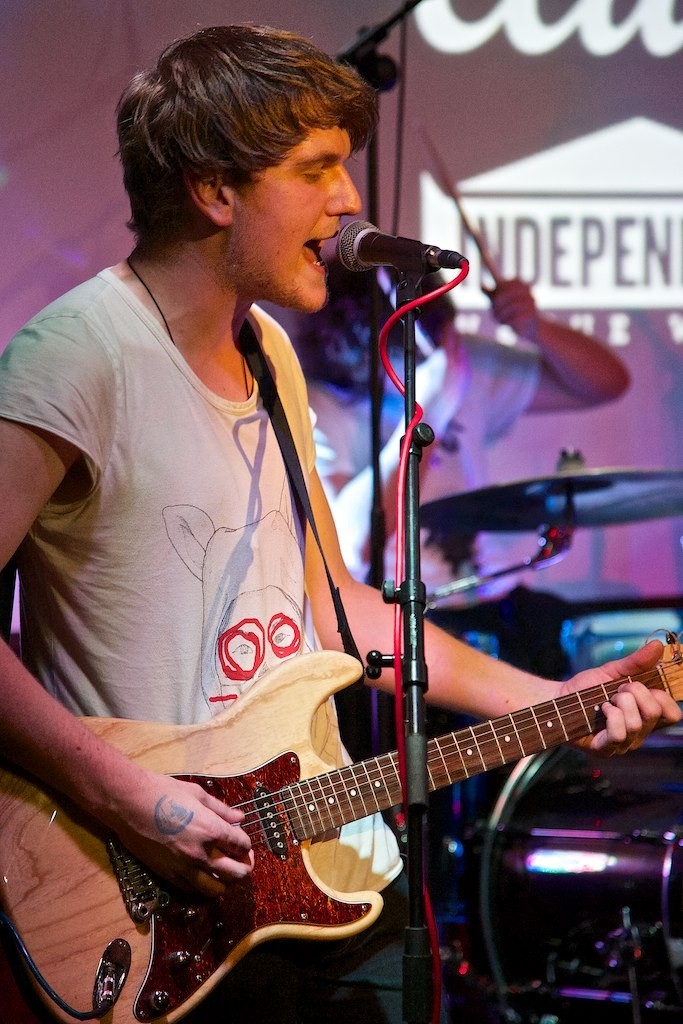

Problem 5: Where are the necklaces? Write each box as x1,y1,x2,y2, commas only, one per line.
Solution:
125,257,250,401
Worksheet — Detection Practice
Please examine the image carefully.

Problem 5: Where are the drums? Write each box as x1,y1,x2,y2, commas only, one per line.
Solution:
479,729,683,1024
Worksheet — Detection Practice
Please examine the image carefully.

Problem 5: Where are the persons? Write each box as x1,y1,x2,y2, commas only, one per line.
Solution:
0,26,683,1024
289,250,631,611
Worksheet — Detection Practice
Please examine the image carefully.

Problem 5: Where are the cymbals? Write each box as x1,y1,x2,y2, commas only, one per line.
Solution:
418,453,683,534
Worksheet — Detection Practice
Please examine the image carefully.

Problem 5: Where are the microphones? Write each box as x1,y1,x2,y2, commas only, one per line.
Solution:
335,220,469,272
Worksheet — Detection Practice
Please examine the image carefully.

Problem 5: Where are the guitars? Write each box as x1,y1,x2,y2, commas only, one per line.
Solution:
0,623,683,1024
